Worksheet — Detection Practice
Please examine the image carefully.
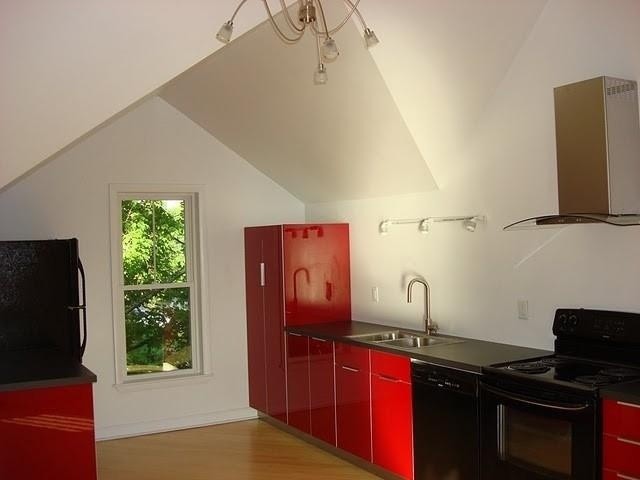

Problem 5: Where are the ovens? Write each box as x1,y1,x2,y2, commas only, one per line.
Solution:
479,376,600,471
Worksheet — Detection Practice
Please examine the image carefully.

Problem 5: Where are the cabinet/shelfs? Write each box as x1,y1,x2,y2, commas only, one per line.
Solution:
245,223,349,419
0,366,99,480
287,332,336,445
604,401,640,480
336,342,414,479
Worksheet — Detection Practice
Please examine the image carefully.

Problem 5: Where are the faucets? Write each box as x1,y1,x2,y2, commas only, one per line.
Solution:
406,278,439,337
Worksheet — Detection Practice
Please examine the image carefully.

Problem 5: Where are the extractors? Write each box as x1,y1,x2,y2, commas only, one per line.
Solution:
499,75,639,231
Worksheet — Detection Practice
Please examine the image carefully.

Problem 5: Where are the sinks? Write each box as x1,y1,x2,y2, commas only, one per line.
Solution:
344,329,426,343
380,335,471,349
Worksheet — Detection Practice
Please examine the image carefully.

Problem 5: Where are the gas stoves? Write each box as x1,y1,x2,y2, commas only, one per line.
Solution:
480,353,639,391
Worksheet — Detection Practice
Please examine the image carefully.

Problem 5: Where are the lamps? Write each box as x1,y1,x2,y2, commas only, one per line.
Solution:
378,216,485,235
214,0,382,85
288,226,326,239
